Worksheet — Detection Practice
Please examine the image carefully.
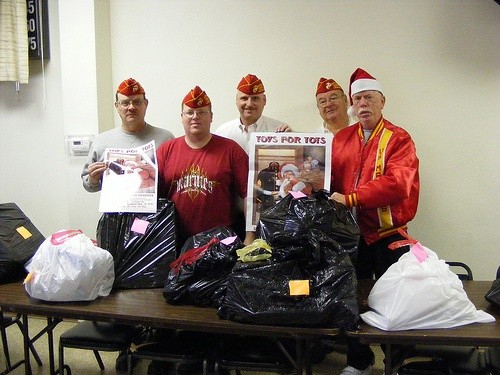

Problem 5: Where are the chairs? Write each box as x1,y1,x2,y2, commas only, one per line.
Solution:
0,261,500,375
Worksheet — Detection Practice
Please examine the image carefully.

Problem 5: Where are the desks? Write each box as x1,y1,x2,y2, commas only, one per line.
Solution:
0,273,500,375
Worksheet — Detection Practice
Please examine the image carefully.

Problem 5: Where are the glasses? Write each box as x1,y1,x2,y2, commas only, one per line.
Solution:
117,99,145,109
181,107,212,119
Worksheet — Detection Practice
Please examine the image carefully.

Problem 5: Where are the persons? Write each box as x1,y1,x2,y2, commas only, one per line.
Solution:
148,86,248,375
80,78,175,374
315,78,356,139
329,68,420,375
216,73,292,242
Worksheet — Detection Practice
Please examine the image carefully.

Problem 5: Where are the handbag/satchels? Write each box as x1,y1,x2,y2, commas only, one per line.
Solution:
23,228,115,302
358,229,495,331
0,202,47,285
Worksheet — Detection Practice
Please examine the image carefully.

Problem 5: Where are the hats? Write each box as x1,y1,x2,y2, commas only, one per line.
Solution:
237,74,265,95
346,68,383,116
315,77,343,96
182,85,211,109
117,77,145,96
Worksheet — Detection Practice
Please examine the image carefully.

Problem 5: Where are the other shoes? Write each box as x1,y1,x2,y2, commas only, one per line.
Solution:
146,359,174,375
383,361,406,375
115,351,140,375
341,365,373,375
